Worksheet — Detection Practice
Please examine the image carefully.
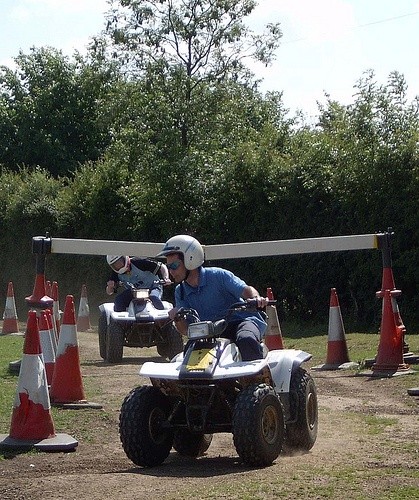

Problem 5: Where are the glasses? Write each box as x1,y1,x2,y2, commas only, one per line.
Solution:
165,258,183,269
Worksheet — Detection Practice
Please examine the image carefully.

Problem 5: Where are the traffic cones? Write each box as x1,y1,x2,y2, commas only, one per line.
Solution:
40,310,57,387
263,287,285,350
50,281,60,334
46,279,52,297
0,309,80,449
311,288,360,370
76,284,93,332
49,294,103,409
2,281,25,336
357,290,417,377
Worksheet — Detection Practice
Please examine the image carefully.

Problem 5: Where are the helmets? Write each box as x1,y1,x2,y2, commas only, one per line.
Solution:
107,254,130,275
155,235,204,270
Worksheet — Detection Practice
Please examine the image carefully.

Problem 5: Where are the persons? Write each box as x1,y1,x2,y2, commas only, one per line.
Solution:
106,250,173,311
156,235,270,361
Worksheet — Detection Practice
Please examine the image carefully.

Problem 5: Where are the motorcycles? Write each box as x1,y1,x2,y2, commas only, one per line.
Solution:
119,299,320,468
97,279,185,362
